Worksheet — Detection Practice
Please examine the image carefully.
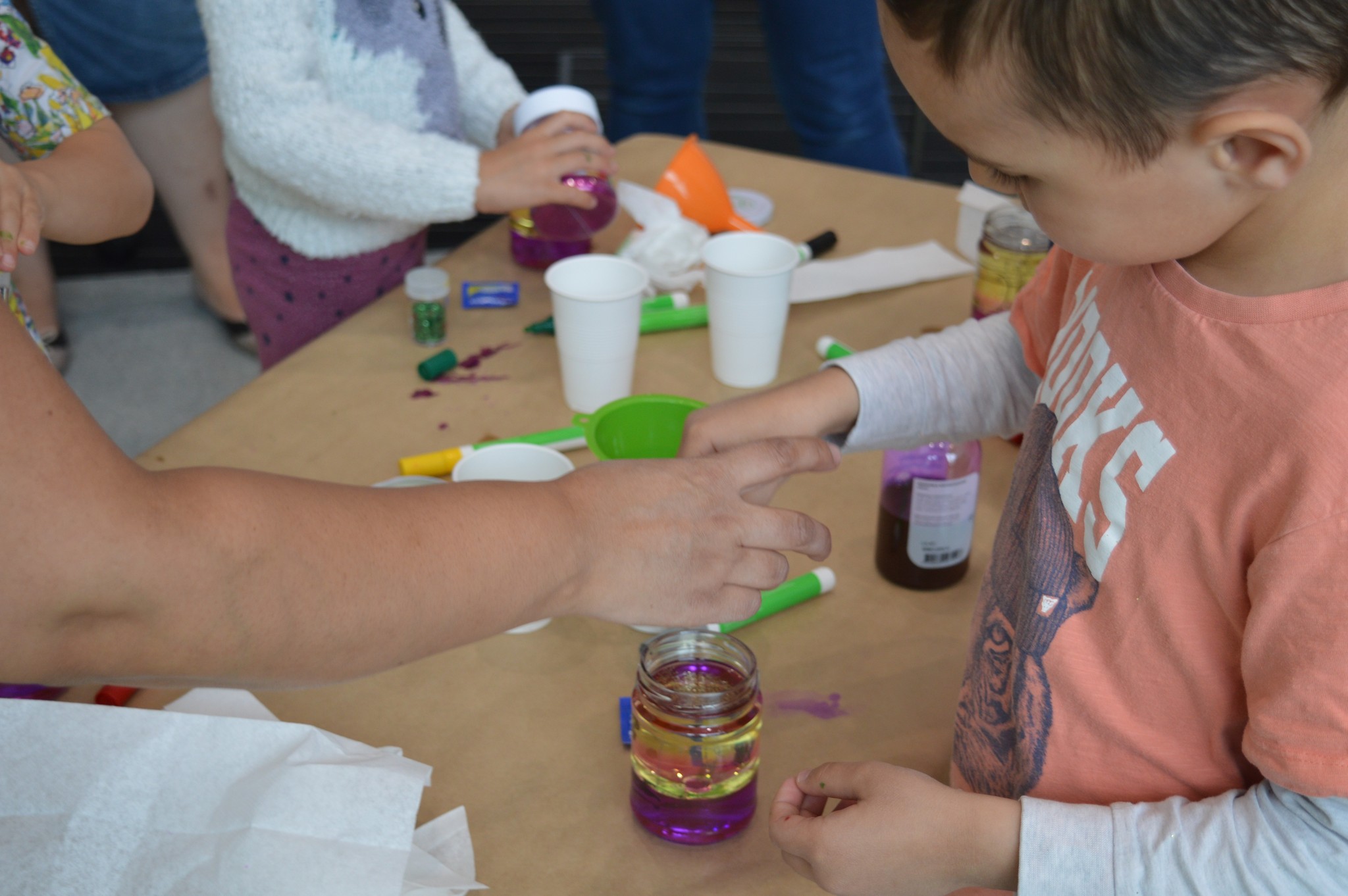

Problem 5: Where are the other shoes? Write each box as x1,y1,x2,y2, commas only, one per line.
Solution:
35,325,70,374
193,288,256,355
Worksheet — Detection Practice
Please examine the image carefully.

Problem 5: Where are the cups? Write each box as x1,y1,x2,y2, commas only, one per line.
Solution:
366,472,445,491
545,253,648,413
451,443,576,636
700,231,803,387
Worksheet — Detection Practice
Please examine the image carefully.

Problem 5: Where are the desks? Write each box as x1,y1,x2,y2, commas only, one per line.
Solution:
62,133,1023,896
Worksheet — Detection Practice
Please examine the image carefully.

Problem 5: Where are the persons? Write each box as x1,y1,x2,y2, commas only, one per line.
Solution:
677,0,1345,894
4,2,838,690
1,4,260,377
194,2,615,374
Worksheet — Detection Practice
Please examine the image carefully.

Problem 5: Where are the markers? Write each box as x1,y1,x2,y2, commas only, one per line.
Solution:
707,567,839,635
814,336,853,359
91,686,137,708
794,227,837,264
400,423,588,478
525,290,691,336
639,304,710,333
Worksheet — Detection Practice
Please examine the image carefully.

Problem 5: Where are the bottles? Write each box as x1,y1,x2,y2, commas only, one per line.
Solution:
875,436,983,591
509,210,591,271
970,207,1052,323
513,86,617,240
629,630,762,844
405,268,448,343
